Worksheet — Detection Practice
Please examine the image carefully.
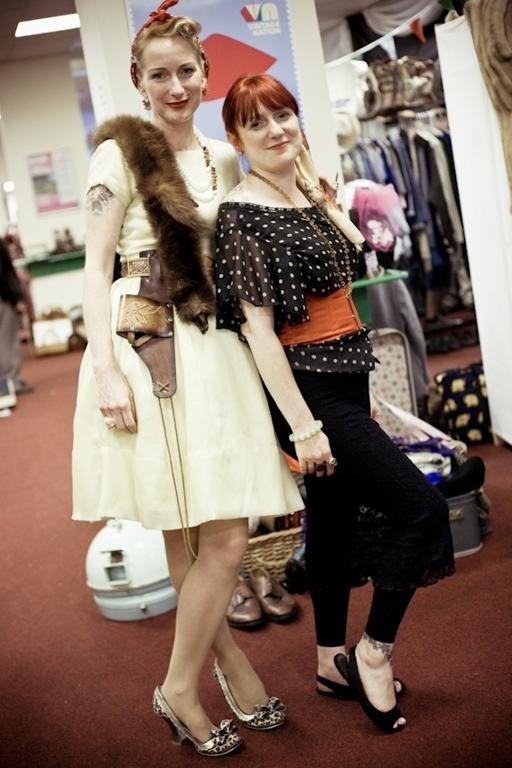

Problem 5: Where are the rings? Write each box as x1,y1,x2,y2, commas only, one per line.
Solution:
328,457,338,467
104,417,118,430
314,462,325,471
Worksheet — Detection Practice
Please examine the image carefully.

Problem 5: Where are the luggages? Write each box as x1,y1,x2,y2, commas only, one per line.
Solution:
369,327,485,560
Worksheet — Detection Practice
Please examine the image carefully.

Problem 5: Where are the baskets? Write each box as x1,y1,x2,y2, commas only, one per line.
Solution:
239,525,306,585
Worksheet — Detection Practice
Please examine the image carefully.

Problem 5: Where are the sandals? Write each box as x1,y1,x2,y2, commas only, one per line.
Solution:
333,644,407,734
315,672,405,700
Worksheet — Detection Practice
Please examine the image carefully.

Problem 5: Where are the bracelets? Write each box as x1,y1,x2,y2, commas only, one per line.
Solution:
288,418,323,443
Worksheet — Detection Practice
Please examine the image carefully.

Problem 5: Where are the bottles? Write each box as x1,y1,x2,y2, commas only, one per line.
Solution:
55,231,64,250
65,229,76,249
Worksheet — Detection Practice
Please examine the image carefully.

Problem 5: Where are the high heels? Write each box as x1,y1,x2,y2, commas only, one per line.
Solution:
152,682,243,758
212,657,288,731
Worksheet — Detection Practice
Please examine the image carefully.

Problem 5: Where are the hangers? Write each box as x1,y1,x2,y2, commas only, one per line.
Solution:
355,108,450,144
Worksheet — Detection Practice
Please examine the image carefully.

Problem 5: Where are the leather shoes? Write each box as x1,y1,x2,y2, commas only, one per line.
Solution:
248,566,298,624
226,575,268,632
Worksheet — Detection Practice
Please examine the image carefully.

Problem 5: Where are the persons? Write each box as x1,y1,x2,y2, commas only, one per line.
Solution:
69,4,304,754
0,237,34,393
213,75,452,734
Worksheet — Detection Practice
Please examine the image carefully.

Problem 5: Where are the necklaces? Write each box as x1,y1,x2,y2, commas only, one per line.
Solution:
246,169,354,299
166,135,218,203
